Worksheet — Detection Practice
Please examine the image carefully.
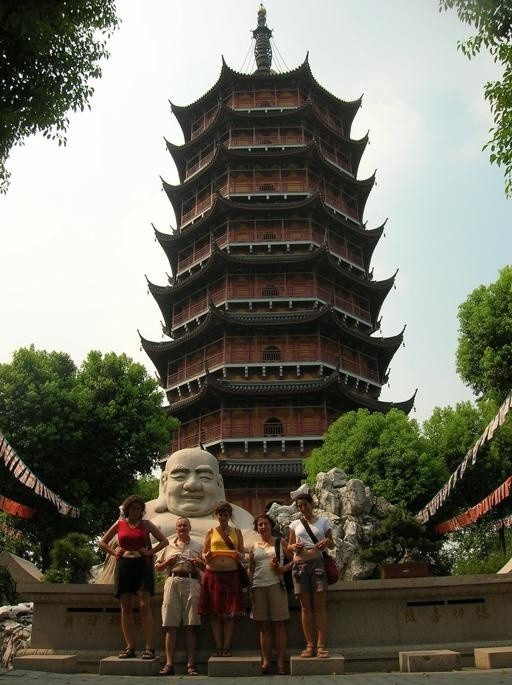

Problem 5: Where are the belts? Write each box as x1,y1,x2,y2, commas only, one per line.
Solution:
168,572,199,580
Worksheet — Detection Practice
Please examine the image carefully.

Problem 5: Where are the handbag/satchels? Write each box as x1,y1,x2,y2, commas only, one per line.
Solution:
215,527,252,589
299,518,340,585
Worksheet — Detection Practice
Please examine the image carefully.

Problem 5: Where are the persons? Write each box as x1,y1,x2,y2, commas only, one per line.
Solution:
287,494,335,657
98,495,169,660
246,514,294,674
154,517,205,676
199,501,243,657
92,448,261,589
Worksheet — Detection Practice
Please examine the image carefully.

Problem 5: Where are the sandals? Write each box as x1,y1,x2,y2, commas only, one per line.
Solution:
277,667,286,675
212,648,222,658
301,645,315,658
275,536,295,594
144,649,154,660
264,666,273,675
161,665,176,676
222,649,232,658
317,646,330,658
119,649,135,659
186,665,200,677
